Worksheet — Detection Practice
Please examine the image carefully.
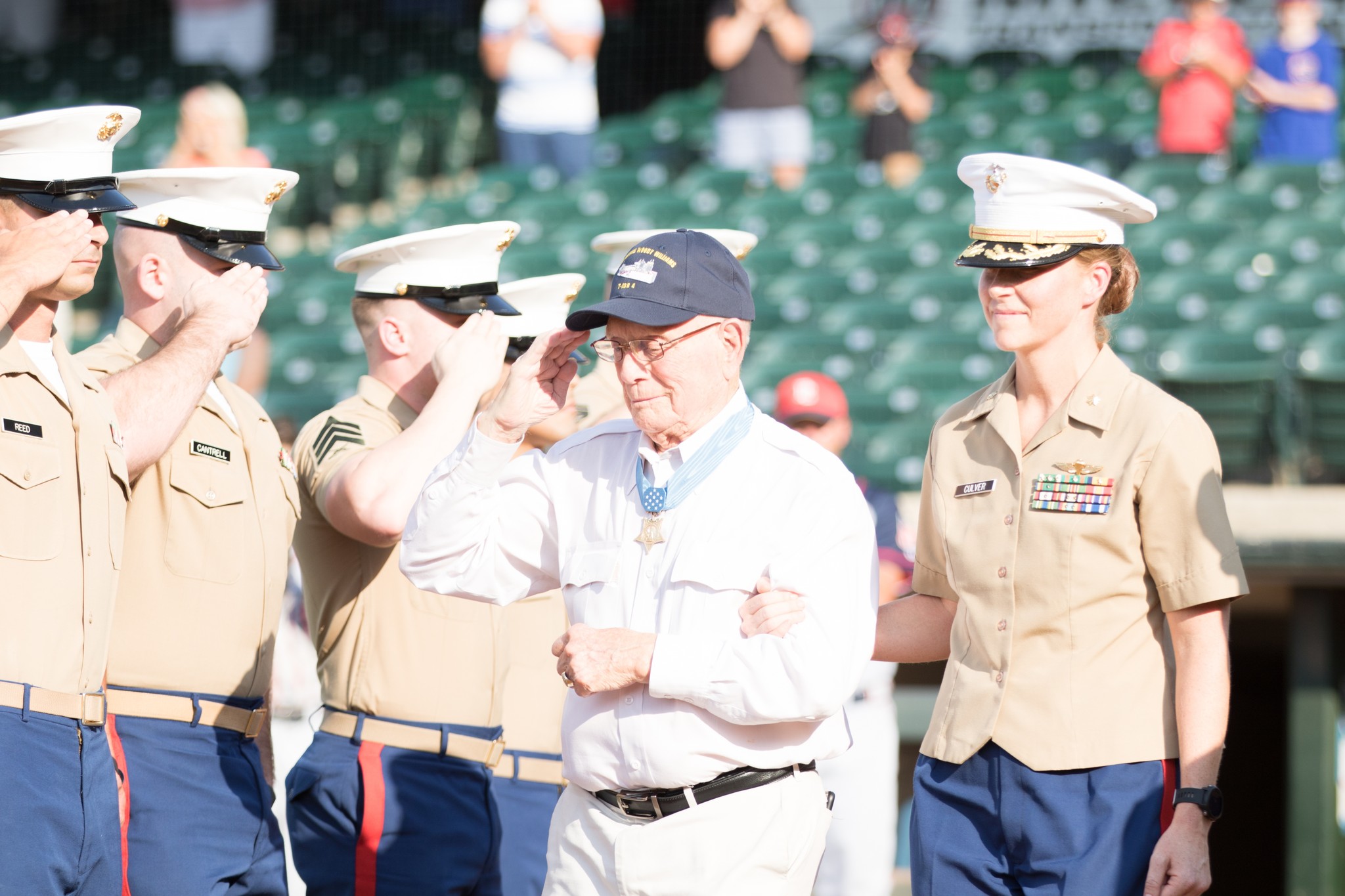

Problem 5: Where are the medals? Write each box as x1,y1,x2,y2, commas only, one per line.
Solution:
633,507,666,555
1031,459,1114,515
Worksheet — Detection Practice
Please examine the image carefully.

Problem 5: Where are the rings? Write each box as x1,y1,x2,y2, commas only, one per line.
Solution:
561,671,575,687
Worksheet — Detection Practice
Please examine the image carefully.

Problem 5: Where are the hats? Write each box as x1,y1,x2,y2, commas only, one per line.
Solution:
954,152,1157,267
776,371,848,429
0,105,141,214
591,228,758,277
334,220,521,318
565,228,755,331
494,273,586,340
109,166,300,271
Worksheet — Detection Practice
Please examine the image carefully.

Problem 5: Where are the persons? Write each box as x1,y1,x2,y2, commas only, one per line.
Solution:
477,0,606,184
849,2,941,192
1241,0,1343,162
0,76,919,896
72,167,304,896
1136,0,1255,155
737,153,1252,896
703,0,815,194
397,230,879,896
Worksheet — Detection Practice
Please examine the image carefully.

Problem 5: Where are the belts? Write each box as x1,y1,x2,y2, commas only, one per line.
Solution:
319,709,507,768
587,760,816,819
0,681,106,727
104,688,267,739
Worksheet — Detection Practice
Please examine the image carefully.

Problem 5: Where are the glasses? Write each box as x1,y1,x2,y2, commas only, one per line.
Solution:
592,320,726,361
505,336,535,361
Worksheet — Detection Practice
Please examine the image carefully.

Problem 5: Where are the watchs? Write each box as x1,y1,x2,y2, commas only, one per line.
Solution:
1172,784,1224,823
